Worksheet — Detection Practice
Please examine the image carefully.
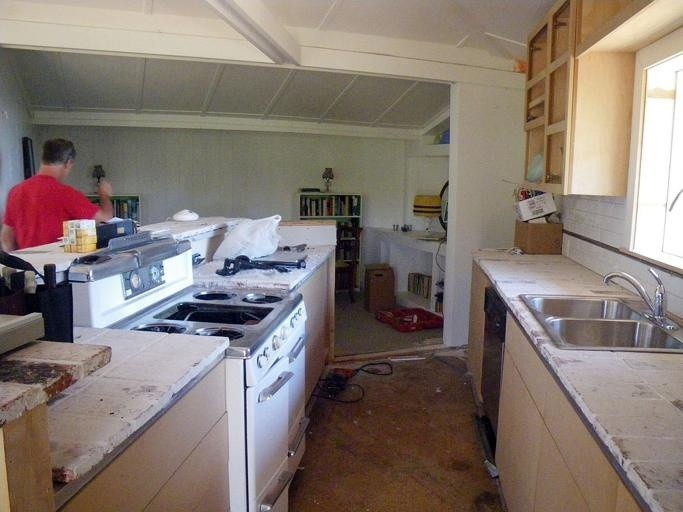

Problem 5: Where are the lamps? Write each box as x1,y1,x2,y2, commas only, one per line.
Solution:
321,167,334,192
91,165,106,194
414,195,443,240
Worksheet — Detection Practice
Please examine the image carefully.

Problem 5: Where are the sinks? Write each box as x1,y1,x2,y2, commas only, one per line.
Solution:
542,318,683,352
520,293,653,319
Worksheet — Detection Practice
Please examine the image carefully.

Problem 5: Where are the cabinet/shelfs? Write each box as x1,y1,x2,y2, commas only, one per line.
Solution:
293,191,364,294
576,1,681,59
298,250,334,408
467,261,504,405
522,1,634,196
57,359,231,512
85,194,141,227
493,308,640,511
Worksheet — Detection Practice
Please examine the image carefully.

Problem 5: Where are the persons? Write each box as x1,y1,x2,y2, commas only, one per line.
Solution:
0,138,114,253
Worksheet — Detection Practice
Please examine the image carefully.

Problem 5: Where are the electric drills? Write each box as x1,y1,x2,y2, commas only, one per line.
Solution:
325,368,352,398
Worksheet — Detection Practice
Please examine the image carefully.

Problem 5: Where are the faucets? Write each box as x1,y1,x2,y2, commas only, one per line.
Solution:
604,266,665,319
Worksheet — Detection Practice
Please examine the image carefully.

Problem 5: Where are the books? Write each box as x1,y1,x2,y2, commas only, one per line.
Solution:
301,196,360,262
408,273,432,299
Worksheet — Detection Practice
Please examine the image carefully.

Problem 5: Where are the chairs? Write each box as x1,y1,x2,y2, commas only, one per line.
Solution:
335,225,364,304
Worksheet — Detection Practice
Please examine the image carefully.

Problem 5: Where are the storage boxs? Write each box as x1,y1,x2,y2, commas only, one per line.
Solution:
364,264,396,313
513,191,562,254
